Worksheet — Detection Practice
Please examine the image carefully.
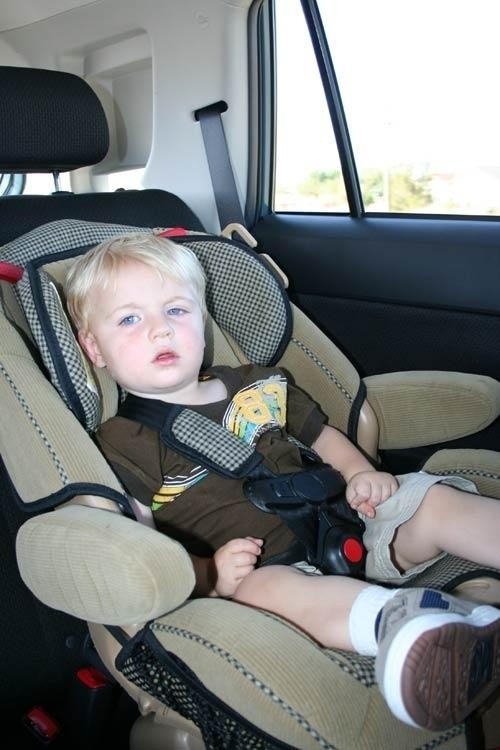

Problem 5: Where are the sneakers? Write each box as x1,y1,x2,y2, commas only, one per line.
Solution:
377,587,499,729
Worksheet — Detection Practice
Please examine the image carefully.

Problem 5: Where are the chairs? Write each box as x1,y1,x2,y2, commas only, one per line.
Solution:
0,63,499,747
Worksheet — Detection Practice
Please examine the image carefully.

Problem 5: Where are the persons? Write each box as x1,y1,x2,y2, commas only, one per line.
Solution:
62,233,500,731
